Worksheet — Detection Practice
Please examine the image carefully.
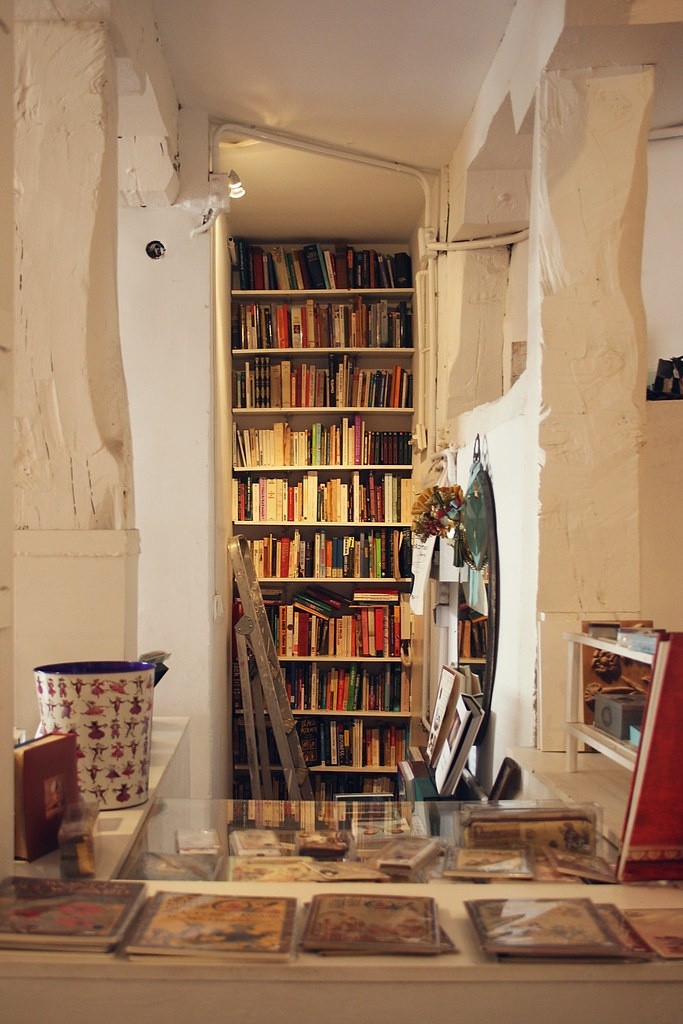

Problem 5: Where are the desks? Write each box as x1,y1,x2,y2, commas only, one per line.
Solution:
3,714,682,1024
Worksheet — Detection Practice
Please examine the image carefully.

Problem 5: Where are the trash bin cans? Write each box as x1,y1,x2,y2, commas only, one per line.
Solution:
32,660,157,811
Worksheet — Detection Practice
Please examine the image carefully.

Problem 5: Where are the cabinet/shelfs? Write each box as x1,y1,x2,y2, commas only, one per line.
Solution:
222,240,414,774
563,631,658,773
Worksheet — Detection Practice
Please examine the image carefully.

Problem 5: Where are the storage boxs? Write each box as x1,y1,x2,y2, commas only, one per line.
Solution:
591,695,645,741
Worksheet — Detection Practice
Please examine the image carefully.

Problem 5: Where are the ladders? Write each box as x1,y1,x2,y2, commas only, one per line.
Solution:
226,534,315,802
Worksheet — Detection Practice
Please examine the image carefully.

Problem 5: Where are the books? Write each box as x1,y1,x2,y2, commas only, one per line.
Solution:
238,240,413,290
0,831,683,961
229,799,410,832
233,663,401,712
240,716,409,766
616,633,683,879
14,733,81,861
232,354,413,408
236,774,395,800
232,415,411,467
398,615,488,803
231,297,412,349
247,527,410,578
238,470,413,522
234,585,413,656
